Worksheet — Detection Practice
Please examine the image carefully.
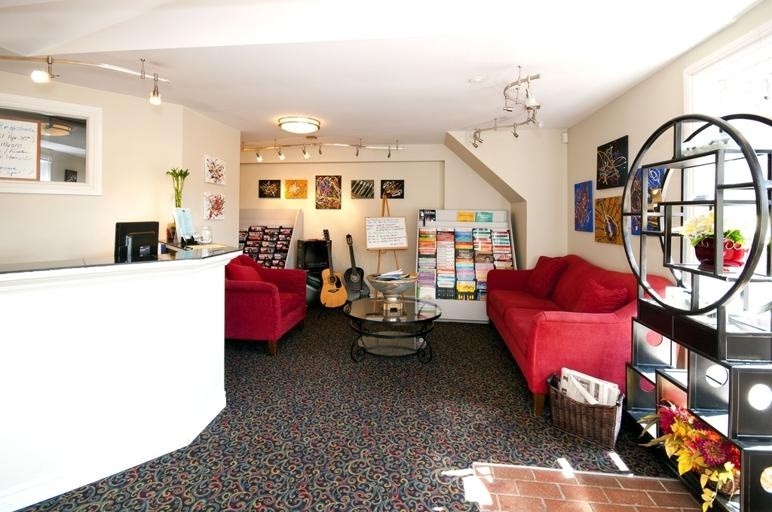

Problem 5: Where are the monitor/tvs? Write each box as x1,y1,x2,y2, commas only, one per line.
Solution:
114,221,159,263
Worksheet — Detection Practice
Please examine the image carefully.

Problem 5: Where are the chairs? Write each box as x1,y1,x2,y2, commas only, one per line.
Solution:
225,254,308,356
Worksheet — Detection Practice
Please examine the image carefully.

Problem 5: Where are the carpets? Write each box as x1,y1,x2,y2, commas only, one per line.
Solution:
470,460,710,511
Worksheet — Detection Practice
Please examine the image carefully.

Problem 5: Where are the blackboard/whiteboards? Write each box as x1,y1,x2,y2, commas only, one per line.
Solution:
0,115,41,181
364,216,409,251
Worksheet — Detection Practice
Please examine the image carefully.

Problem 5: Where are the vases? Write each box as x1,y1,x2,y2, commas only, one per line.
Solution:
694,239,746,271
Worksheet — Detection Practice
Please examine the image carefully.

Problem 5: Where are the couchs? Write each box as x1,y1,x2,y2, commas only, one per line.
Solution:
486,251,681,416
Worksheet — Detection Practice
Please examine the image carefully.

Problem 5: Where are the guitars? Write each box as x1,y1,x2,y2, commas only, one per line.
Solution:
320,230,347,307
344,234,370,302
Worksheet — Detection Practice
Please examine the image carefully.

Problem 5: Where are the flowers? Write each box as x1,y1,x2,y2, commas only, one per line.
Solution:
638,401,742,512
679,209,744,248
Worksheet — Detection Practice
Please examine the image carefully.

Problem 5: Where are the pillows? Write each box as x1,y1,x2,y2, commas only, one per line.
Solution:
524,256,567,299
227,264,264,281
573,279,627,312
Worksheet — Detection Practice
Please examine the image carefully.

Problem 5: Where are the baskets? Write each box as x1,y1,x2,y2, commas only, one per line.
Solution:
542,368,628,453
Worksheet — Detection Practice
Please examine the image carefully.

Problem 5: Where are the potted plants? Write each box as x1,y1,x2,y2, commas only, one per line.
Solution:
167,167,190,207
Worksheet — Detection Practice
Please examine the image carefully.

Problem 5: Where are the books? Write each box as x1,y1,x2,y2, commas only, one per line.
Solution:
377,268,417,282
558,366,621,408
417,208,514,301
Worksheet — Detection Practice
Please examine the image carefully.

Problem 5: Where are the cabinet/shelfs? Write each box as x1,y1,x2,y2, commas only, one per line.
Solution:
414,208,518,327
623,110,771,511
239,208,304,271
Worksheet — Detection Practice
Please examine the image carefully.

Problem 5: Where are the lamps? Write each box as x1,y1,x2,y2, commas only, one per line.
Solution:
279,116,321,136
468,64,543,150
240,137,408,160
41,121,73,137
28,55,163,109
